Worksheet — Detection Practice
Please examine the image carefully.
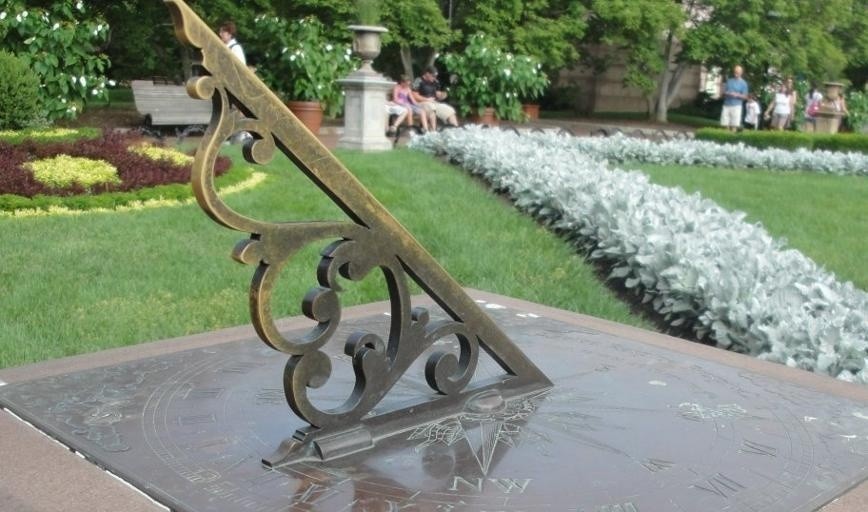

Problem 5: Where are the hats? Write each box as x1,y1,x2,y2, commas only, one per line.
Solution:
424,66,438,75
823,81,844,86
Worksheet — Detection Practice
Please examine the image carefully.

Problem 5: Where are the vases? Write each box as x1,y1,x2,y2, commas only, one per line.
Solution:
285,100,323,136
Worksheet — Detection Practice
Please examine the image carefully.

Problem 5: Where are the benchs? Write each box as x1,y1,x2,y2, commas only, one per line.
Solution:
131,80,215,141
387,111,439,136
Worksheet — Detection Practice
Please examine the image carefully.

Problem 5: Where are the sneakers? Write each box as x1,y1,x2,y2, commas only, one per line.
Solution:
387,125,438,138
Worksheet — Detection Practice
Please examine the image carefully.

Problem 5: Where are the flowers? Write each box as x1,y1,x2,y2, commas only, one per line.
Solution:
243,1,362,119
435,29,551,127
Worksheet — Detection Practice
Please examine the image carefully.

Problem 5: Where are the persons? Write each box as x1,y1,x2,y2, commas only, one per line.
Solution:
386,96,409,135
804,80,822,132
218,21,246,147
743,94,761,130
719,65,749,132
392,73,437,132
411,65,458,127
786,78,797,129
764,82,793,130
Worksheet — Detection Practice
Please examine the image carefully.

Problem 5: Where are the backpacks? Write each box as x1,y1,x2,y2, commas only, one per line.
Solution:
807,101,819,117
